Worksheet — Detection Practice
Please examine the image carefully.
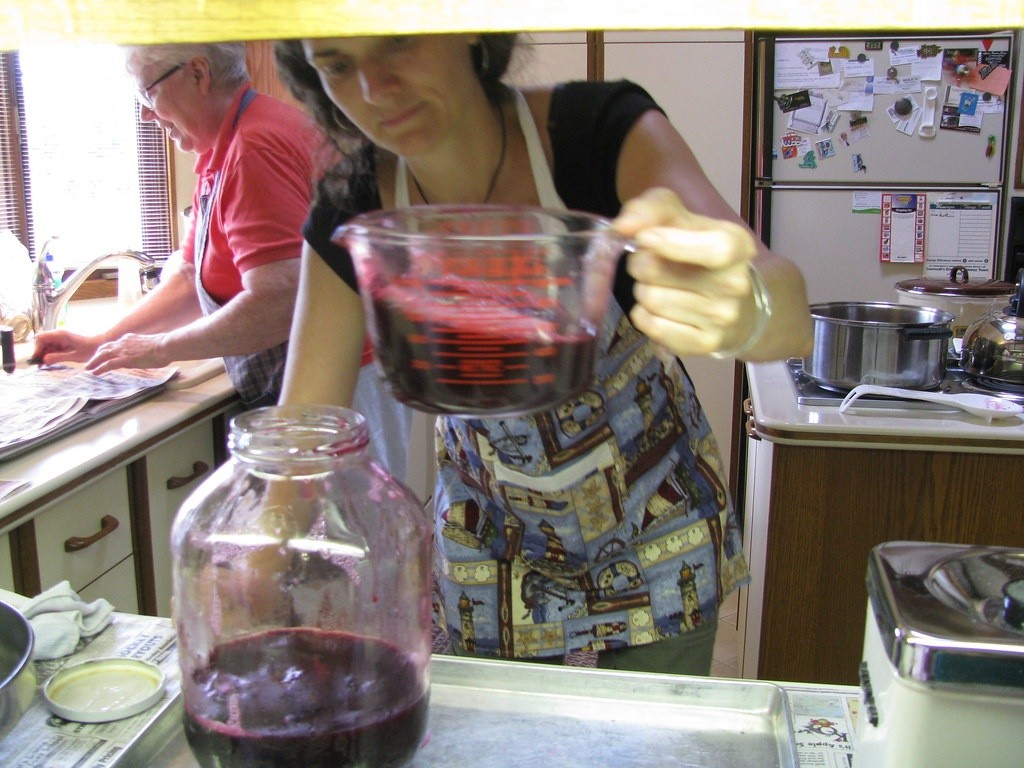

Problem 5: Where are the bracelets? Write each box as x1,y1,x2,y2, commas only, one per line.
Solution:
711,259,774,361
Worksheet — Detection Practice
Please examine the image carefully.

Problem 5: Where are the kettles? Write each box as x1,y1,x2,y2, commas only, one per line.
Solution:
963,268,1024,386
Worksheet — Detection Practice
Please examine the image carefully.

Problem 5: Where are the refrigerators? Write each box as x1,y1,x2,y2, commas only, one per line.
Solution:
740,29,1023,303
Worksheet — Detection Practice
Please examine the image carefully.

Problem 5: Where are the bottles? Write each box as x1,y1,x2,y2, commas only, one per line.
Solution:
170,404,433,768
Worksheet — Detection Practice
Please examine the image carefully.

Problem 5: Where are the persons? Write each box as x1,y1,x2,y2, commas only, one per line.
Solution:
199,33,817,673
31,42,409,636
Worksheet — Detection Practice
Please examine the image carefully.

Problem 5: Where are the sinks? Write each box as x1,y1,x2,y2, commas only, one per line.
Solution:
163,356,226,389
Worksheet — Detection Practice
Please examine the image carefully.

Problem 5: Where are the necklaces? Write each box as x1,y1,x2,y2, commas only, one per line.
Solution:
408,102,507,237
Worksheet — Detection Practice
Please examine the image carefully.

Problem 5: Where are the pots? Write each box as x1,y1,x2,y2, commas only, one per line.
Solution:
804,299,956,393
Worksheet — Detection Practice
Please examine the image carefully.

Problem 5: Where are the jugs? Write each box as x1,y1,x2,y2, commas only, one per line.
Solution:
328,203,648,420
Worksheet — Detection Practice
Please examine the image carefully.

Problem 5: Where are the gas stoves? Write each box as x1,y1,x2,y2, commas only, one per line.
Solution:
784,351,1022,411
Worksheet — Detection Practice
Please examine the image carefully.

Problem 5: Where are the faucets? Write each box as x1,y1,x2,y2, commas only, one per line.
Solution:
30,234,156,336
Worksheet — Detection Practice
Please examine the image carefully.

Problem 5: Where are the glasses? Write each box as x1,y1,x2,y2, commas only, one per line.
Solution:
135,63,186,108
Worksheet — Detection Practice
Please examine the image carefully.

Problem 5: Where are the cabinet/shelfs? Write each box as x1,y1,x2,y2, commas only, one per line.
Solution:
0,398,244,618
737,360,1024,688
